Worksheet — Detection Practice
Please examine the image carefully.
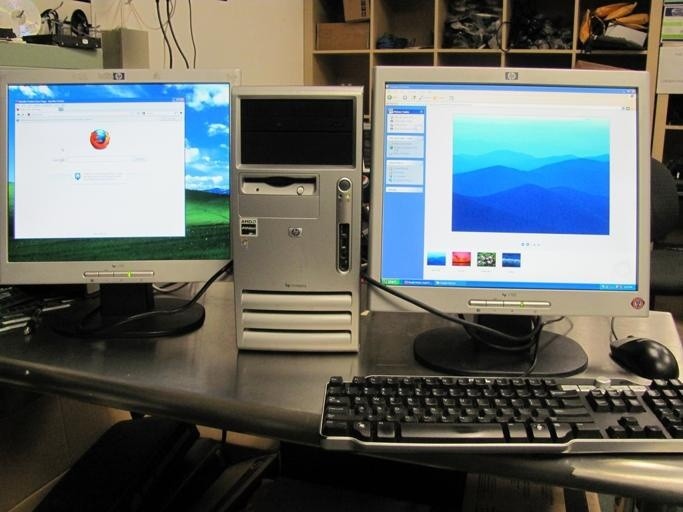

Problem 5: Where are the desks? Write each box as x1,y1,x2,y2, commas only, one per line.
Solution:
0,283,682,512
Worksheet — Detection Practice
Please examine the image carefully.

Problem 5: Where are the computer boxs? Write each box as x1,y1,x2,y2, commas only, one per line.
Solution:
230,85,364,354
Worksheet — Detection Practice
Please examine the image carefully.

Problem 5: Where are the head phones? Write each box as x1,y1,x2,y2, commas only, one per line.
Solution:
41,9,91,35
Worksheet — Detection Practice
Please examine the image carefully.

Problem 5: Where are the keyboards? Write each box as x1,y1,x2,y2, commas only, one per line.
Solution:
317,375,683,463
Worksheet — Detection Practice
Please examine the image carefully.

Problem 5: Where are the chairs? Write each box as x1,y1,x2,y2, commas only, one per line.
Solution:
651,154,682,307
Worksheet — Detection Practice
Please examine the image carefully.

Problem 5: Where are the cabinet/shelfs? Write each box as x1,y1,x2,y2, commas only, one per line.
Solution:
303,0,683,182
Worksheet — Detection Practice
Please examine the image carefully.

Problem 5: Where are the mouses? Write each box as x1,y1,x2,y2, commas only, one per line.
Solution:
610,336,679,379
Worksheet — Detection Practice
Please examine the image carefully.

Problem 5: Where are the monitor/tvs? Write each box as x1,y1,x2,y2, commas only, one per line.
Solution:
1,67,242,338
369,66,651,377
